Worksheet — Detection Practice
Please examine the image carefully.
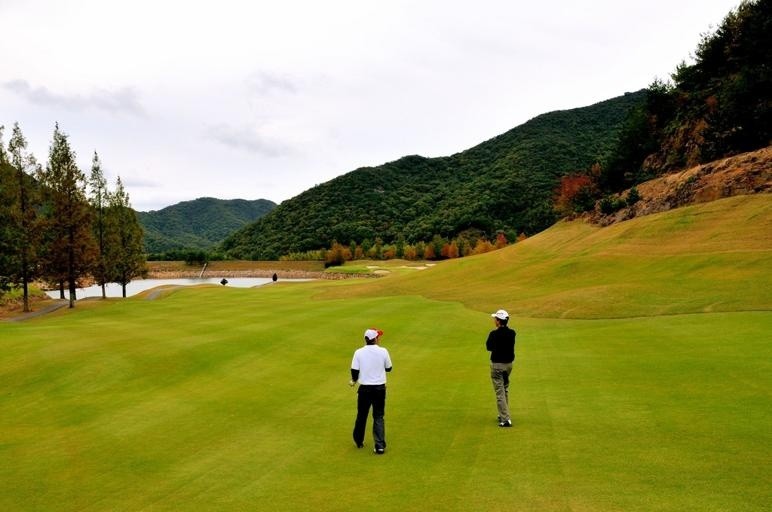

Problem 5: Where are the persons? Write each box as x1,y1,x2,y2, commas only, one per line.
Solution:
349,327,393,454
486,309,516,426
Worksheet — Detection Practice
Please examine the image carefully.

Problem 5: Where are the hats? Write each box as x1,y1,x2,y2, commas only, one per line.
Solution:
490,309,510,321
364,328,383,341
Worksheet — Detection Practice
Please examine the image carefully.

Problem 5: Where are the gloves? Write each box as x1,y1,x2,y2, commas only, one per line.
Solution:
348,380,355,387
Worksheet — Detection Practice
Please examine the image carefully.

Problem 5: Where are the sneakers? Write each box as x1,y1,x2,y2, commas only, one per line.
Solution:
355,441,365,450
372,446,384,454
497,417,511,427
